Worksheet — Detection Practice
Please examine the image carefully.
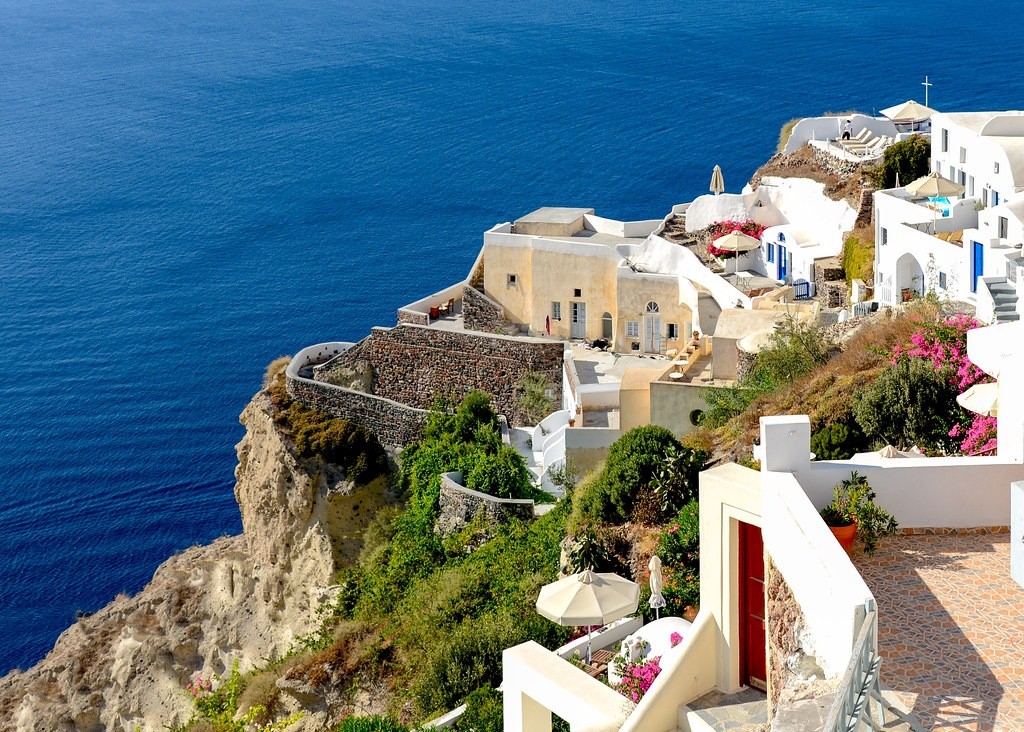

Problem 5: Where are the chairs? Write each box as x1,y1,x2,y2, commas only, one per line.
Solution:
443,298,454,313
573,650,616,677
934,230,963,247
835,127,886,156
748,287,774,298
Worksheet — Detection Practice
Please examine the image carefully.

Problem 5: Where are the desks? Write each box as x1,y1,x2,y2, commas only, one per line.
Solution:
734,271,754,290
669,372,684,382
674,360,690,379
439,307,447,316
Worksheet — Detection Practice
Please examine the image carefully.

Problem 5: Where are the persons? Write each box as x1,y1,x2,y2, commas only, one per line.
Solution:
842,119,853,140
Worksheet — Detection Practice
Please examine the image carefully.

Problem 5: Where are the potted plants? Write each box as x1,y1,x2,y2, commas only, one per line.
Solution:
818,470,901,558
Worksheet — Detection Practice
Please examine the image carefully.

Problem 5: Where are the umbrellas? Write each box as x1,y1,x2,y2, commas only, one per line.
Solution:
851,445,926,461
709,165,724,196
713,229,761,272
956,381,997,417
905,169,964,233
536,570,641,664
879,100,940,131
647,556,666,619
